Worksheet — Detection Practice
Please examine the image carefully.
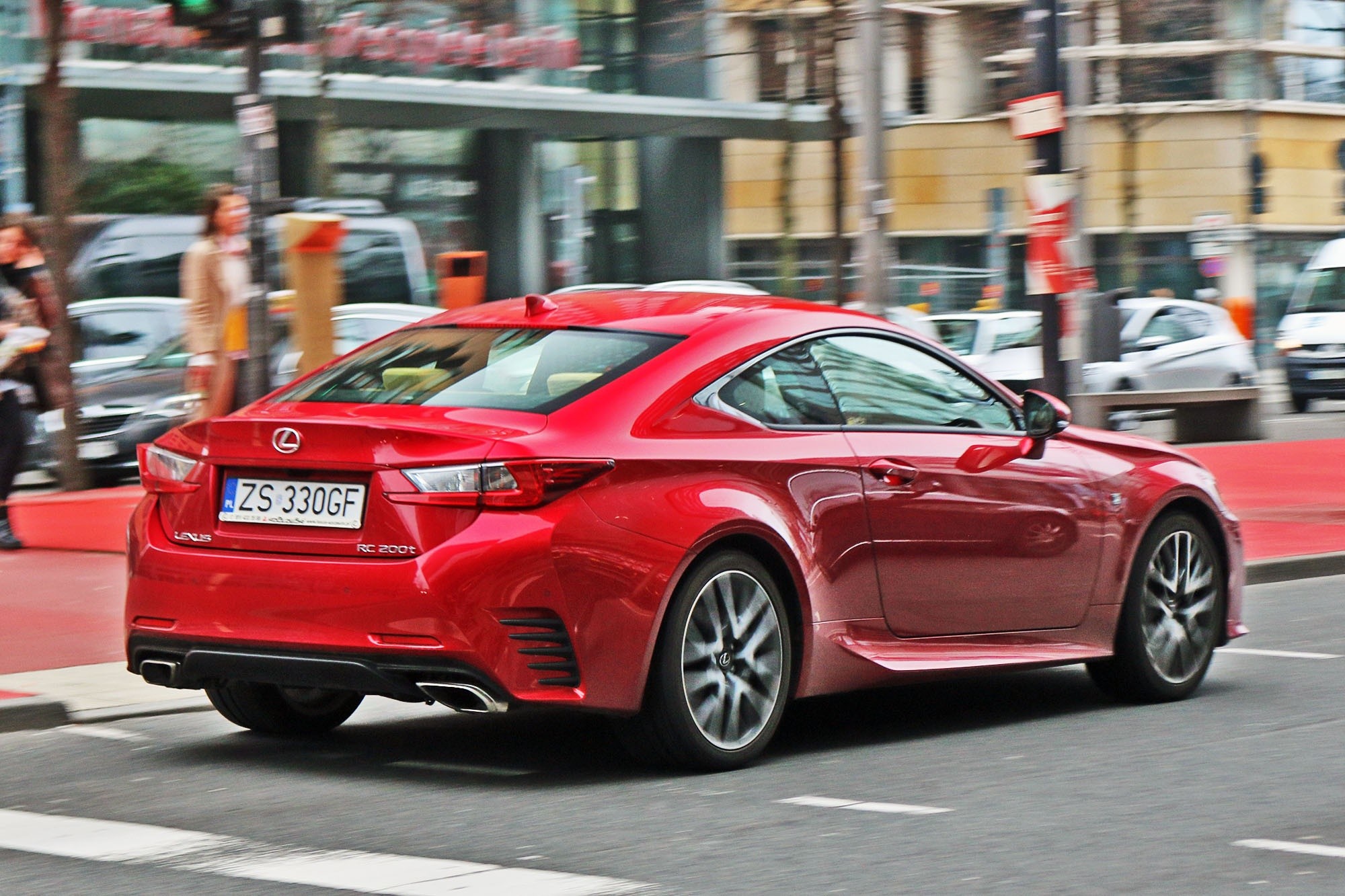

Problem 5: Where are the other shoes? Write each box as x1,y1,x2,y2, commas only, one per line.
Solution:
0,518,23,550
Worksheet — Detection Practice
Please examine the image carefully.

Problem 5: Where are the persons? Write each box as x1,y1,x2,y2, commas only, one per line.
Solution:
0,213,61,549
177,184,251,420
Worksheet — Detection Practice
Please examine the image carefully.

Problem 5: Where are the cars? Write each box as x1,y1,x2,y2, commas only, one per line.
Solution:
920,236,1263,435
119,287,1252,771
2,194,450,493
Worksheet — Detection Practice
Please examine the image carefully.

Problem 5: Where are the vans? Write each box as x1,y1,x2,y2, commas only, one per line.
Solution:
1272,238,1345,414
